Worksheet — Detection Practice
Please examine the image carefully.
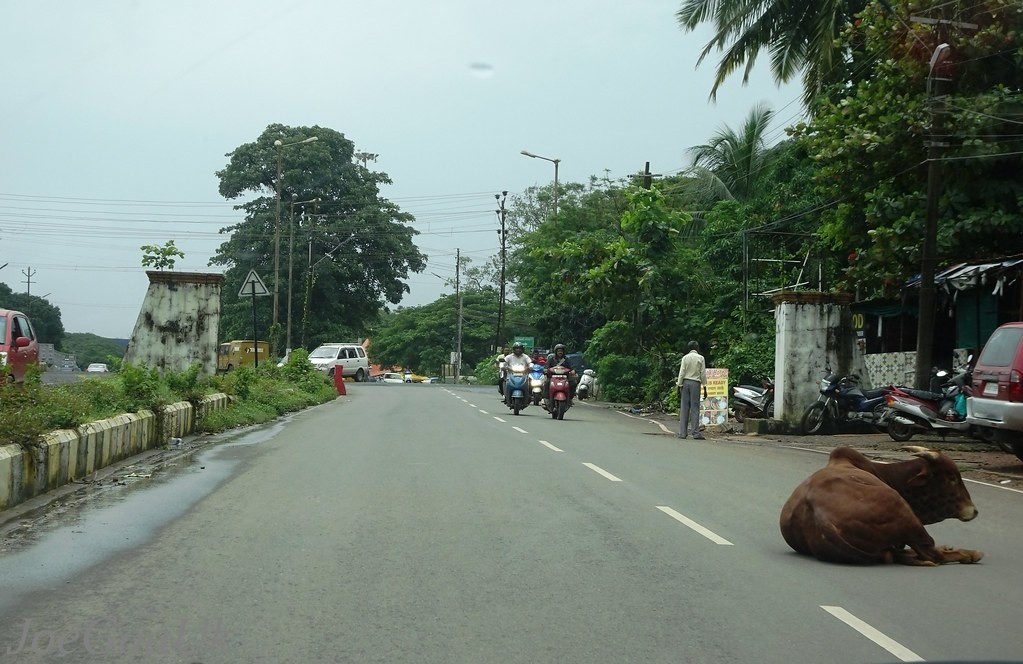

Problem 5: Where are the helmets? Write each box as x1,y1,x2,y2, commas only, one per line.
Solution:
513,342,524,353
554,344,565,357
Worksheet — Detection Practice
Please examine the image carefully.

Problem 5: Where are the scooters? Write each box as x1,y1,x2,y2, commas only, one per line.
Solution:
498,358,544,416
882,350,996,444
575,365,597,400
543,365,573,420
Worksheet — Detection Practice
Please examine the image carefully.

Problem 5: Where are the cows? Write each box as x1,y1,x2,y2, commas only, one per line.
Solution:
780,446,985,566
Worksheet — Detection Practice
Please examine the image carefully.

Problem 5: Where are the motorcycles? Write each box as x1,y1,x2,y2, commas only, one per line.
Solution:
799,364,908,436
732,376,774,423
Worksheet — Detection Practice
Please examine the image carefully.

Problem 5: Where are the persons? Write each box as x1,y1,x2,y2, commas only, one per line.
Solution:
677,342,708,439
499,342,534,407
541,345,577,409
531,351,545,365
496,346,510,393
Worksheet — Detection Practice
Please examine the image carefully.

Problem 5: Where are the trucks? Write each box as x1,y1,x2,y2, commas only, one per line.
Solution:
218,339,269,374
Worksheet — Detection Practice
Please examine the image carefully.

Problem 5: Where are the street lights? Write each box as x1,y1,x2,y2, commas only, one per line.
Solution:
520,151,562,213
272,137,318,326
286,192,322,361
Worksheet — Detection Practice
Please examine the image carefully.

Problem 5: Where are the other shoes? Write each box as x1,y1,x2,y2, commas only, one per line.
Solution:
694,438,706,440
501,395,505,402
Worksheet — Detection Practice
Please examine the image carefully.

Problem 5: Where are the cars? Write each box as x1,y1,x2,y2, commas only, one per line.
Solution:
87,363,109,373
0,308,39,386
380,367,441,384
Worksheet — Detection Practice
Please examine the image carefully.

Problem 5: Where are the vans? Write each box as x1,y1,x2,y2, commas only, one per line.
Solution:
308,343,370,382
966,321,1023,464
